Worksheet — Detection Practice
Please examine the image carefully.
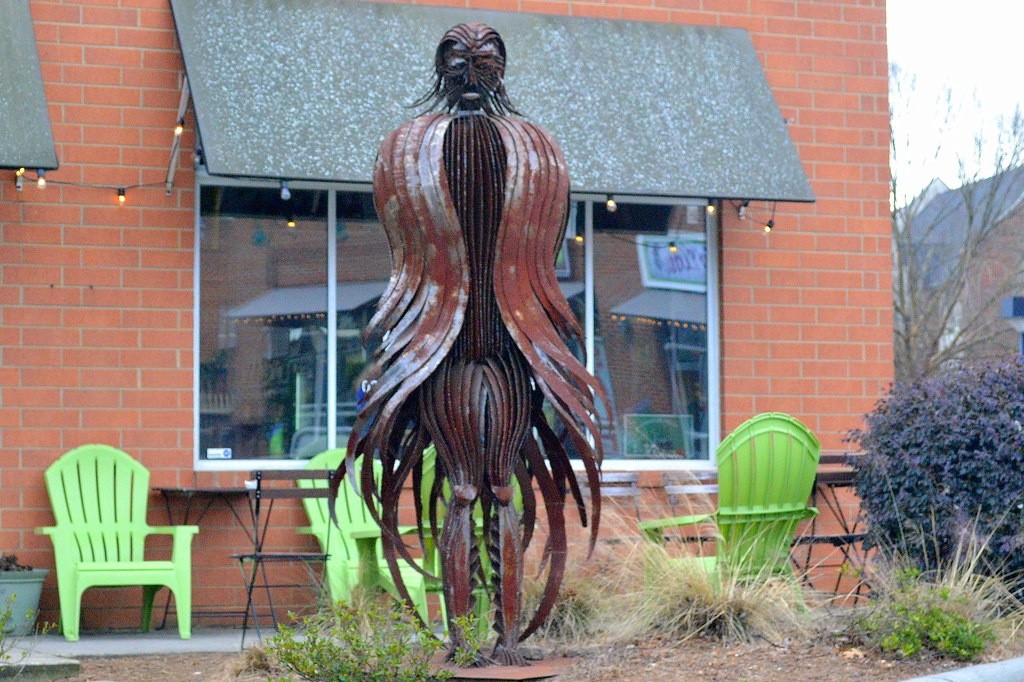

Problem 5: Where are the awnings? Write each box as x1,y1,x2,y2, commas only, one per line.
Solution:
167,1,817,204
609,289,706,327
556,281,587,302
0,0,59,171
220,281,391,318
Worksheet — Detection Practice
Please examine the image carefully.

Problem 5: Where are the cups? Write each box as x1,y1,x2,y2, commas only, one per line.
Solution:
244,479,257,490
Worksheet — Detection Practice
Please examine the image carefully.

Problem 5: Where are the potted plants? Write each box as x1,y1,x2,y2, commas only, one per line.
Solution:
0,552,51,638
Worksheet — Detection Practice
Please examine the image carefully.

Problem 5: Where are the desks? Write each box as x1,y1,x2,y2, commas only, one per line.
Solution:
152,482,282,633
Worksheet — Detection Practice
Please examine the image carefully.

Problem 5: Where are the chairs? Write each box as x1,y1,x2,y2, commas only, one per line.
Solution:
227,469,343,651
295,447,428,631
349,431,535,638
570,468,674,602
663,467,721,553
31,437,201,642
784,452,886,608
638,409,824,626
